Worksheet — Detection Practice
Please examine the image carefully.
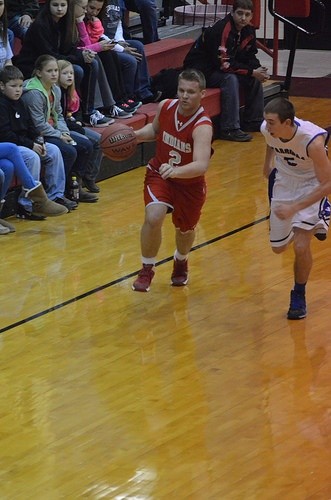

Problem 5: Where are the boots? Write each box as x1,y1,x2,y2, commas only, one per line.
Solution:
0,199,15,234
22,180,69,217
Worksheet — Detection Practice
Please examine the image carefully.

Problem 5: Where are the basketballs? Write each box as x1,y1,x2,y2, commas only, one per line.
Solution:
101,124,137,161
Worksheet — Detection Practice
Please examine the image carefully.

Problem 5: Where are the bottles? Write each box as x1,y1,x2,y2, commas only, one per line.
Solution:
35,132,44,147
70,177,79,204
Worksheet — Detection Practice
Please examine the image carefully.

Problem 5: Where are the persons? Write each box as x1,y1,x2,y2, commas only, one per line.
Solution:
22,54,99,211
23,1,109,128
74,0,133,124
183,0,270,142
57,59,104,193
0,0,13,72
6,1,33,53
0,65,78,221
125,0,161,43
0,142,69,234
84,0,143,113
127,69,215,292
97,0,162,105
259,97,331,321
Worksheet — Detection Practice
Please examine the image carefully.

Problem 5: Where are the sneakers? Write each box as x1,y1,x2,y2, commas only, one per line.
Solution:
65,186,99,203
110,106,133,119
82,175,100,192
17,203,46,219
83,110,114,127
118,99,142,113
146,91,162,103
170,258,189,285
54,196,78,209
0,224,10,234
241,120,263,132
314,233,327,241
219,126,253,142
132,263,155,292
287,289,308,319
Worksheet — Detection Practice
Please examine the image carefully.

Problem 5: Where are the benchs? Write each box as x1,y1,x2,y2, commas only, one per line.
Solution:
0,37,283,219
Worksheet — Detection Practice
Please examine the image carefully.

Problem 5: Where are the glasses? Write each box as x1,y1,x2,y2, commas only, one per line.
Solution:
75,2,88,10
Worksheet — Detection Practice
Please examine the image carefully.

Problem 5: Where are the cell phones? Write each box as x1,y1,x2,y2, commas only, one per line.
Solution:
109,42,118,45
